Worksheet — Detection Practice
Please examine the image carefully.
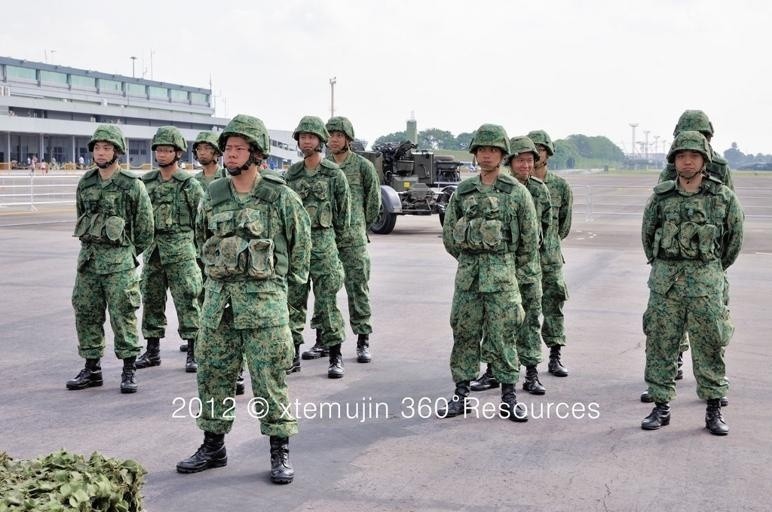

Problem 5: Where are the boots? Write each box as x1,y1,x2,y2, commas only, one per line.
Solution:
66,326,729,485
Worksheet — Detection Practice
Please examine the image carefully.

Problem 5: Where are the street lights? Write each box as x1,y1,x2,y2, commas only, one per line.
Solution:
629,122,668,165
330,76,337,116
129,55,137,77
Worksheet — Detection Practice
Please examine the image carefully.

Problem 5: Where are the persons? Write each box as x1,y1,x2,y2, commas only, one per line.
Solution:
177,114,313,484
30,109,745,436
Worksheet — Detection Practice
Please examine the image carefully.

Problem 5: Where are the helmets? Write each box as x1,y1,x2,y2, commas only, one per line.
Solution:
87,115,355,156
666,110,713,165
468,124,555,166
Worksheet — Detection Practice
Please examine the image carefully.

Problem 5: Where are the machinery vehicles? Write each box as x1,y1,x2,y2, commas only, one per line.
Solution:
368,141,462,234
